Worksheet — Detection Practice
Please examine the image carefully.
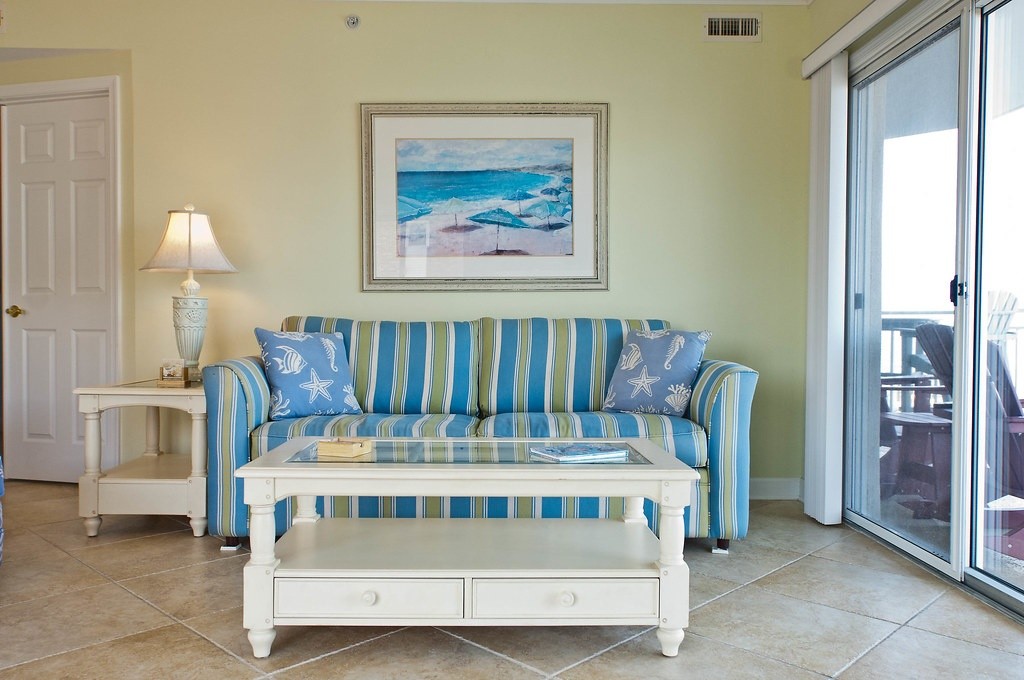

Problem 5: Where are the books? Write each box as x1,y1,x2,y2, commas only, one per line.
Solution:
317,437,372,457
529,444,629,462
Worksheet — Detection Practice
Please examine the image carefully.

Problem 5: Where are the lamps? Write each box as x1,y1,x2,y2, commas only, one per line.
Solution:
139,203,239,383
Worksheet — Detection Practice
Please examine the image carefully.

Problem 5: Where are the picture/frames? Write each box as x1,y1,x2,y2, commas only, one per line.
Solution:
359,101,610,291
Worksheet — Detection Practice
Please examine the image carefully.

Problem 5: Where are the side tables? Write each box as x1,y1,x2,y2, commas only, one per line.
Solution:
73,377,208,537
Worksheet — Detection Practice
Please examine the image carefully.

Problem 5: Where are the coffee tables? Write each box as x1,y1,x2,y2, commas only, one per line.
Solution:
236,435,702,659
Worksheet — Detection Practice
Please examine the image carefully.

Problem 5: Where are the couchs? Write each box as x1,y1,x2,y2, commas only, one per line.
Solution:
203,312,757,554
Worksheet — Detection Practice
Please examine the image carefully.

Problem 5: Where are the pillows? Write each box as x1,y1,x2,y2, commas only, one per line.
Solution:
255,327,362,420
281,316,481,417
602,330,712,419
478,315,673,415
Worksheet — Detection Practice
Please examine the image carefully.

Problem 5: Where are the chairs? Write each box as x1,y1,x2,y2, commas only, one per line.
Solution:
881,323,1024,537
